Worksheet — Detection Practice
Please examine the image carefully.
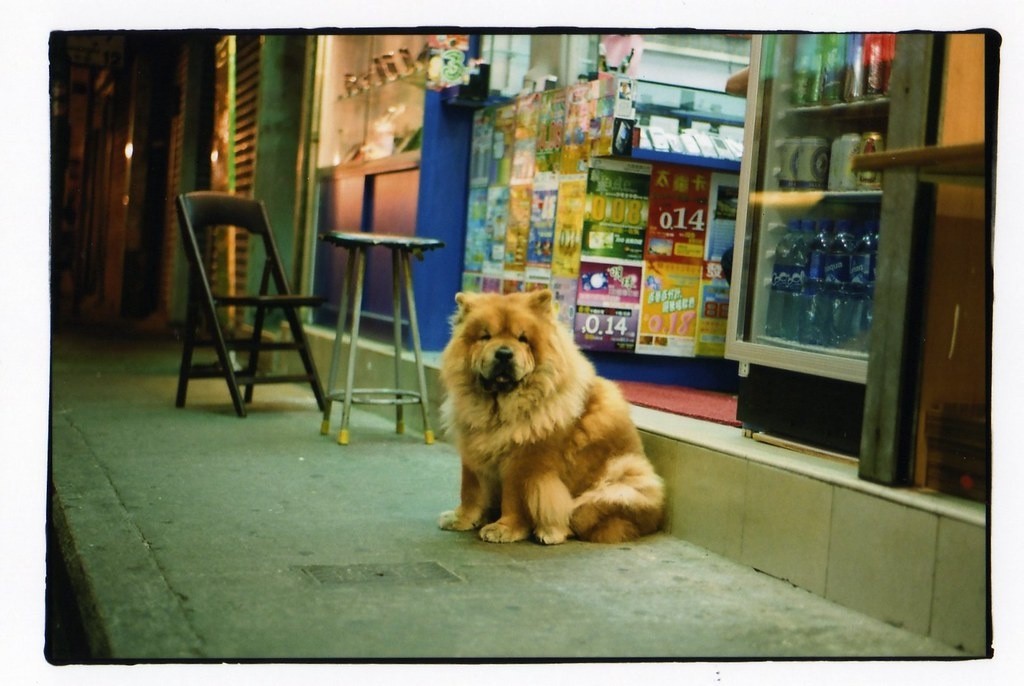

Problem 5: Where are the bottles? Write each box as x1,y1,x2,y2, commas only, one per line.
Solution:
793,34,894,105
765,219,882,349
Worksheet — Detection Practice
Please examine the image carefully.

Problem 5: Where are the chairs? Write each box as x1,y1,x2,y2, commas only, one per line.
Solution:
177,192,325,421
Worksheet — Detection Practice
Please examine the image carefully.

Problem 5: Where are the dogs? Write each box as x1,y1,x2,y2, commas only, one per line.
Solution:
438,288,669,548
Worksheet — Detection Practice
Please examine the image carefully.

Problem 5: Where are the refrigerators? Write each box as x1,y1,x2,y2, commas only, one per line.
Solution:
723,30,899,456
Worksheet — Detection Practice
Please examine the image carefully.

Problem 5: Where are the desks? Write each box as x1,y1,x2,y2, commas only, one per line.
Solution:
320,226,444,446
